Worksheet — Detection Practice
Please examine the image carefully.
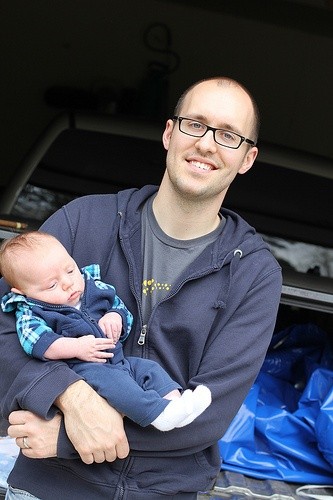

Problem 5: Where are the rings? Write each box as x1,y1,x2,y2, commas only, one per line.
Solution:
23,437,30,449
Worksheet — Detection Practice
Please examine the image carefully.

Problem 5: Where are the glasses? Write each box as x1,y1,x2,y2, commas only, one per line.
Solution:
173,115,258,150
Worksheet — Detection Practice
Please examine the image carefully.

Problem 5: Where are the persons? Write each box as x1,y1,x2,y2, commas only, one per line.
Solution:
0,232,213,432
0,76,283,500
